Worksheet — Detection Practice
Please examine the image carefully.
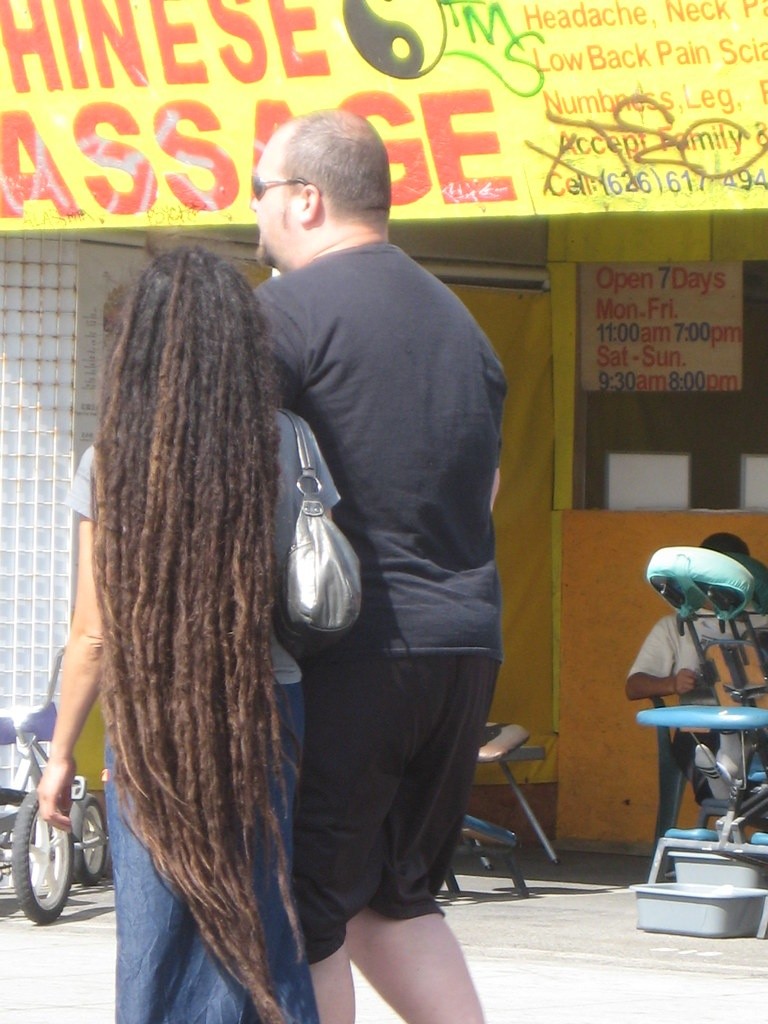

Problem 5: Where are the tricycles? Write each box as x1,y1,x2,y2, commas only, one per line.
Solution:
1,699,110,926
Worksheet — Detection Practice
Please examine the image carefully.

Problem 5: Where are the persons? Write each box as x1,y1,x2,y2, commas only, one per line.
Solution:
249,109,506,1024
624,533,768,831
36,245,342,1024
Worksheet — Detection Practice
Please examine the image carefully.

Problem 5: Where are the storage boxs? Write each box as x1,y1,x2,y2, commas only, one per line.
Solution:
629,850,768,937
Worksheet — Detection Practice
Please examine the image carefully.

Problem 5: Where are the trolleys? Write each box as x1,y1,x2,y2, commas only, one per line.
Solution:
635,546,768,883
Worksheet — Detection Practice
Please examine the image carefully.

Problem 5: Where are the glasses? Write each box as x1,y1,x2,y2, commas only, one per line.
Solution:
249,173,311,201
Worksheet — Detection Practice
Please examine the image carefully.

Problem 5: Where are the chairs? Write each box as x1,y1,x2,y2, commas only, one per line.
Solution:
477,720,560,865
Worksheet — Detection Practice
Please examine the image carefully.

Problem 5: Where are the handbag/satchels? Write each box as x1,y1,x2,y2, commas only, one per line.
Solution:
273,406,364,639
